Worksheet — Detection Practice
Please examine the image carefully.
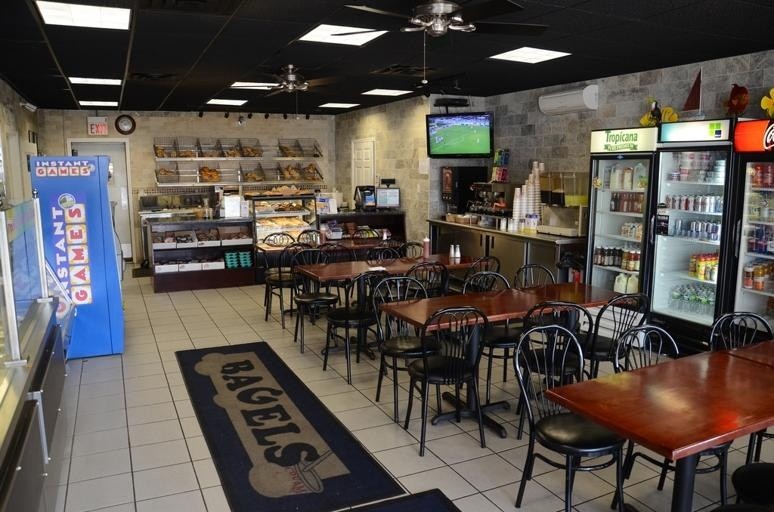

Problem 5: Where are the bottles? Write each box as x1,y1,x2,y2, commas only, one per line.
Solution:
671,282,716,316
594,245,640,271
455,245,461,257
748,224,774,256
449,258,455,265
382,229,388,241
320,229,327,245
744,259,774,291
750,193,774,222
449,244,455,258
621,222,643,241
455,258,461,265
423,234,430,258
688,251,719,282
610,192,645,213
752,165,774,188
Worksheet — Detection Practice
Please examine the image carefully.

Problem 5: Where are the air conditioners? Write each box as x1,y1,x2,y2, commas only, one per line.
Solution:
538,85,599,116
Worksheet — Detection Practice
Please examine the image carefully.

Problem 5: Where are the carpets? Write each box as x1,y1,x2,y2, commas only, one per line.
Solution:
174,341,405,510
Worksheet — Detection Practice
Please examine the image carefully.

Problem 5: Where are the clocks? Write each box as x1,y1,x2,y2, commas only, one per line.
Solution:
115,115,136,135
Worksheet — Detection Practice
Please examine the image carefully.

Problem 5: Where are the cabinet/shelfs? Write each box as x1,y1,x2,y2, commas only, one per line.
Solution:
152,136,325,186
0,325,67,510
145,208,405,294
427,219,560,292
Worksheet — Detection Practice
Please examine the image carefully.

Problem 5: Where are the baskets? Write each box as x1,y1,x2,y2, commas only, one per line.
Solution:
456,216,477,224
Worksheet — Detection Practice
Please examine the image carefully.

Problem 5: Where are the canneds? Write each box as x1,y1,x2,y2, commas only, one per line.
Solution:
675,219,718,240
665,193,724,213
753,163,763,187
764,163,774,187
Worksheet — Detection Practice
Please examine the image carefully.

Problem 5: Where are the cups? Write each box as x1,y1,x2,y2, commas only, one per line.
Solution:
500,161,545,236
285,462,324,496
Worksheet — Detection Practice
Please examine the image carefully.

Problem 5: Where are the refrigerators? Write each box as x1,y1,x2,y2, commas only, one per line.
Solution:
583,112,774,360
28,155,125,360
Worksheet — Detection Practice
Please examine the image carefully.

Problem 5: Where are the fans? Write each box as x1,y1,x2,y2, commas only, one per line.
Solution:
330,0,549,38
223,65,347,98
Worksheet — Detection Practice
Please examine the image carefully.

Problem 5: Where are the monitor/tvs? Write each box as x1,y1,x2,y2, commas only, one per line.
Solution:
376,188,401,210
425,111,495,160
